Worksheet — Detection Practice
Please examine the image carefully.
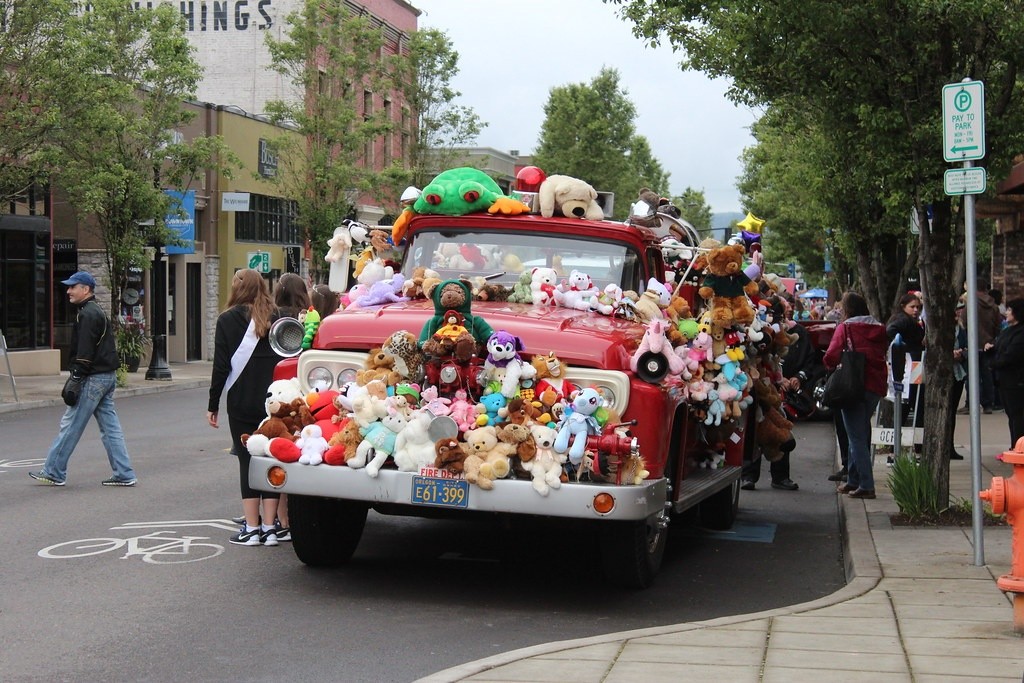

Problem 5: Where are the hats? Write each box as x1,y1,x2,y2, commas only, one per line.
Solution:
61,271,96,287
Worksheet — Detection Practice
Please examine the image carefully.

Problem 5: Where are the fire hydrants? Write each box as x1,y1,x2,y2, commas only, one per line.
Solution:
979,435,1024,638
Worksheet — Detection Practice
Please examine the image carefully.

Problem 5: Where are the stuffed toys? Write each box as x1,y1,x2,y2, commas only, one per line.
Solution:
241,166,798,494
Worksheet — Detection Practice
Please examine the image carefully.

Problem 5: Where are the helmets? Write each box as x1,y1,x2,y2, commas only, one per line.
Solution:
781,384,817,420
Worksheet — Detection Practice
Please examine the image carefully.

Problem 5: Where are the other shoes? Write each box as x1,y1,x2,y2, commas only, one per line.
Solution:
958,406,969,414
232,515,261,524
828,467,847,482
837,484,876,498
887,455,921,467
771,479,799,490
983,407,992,413
740,479,756,490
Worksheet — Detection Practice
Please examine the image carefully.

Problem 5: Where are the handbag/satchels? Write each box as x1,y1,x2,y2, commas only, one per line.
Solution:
823,324,866,407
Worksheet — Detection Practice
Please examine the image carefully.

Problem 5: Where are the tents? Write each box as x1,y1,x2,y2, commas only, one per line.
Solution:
798,288,827,308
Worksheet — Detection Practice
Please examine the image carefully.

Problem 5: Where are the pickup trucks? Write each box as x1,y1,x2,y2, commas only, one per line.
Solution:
248,185,743,586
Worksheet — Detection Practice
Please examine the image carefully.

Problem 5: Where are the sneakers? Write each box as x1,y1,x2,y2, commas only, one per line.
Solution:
29,470,65,485
272,521,291,540
259,527,279,546
102,475,135,486
228,522,260,545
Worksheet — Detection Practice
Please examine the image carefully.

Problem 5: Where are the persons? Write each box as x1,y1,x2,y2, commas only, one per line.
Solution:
741,280,1024,498
205,270,346,547
28,273,137,486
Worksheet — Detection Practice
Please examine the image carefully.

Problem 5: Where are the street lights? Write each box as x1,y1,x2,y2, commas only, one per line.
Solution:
144,130,174,382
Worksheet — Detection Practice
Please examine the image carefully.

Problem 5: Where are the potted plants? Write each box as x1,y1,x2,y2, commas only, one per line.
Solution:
116,328,147,373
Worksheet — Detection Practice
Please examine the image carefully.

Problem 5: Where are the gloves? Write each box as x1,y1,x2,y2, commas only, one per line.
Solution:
61,365,89,407
